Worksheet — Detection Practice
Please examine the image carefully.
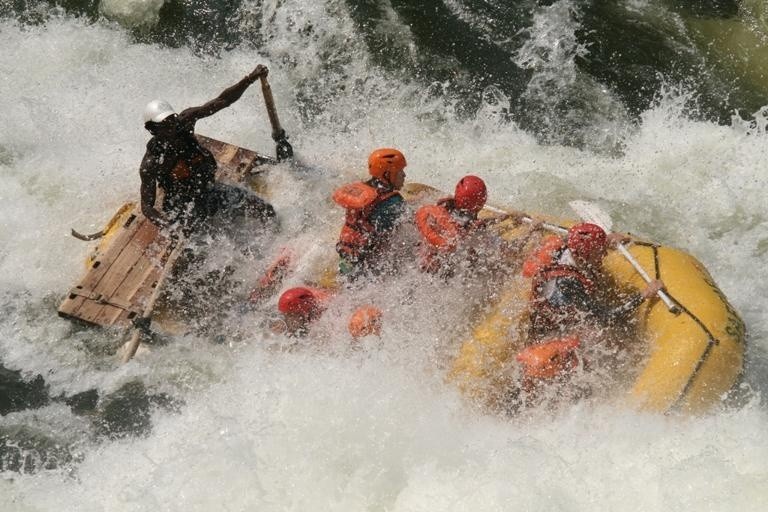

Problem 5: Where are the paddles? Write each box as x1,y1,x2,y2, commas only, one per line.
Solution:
260,77,292,160
404,182,569,235
569,199,678,315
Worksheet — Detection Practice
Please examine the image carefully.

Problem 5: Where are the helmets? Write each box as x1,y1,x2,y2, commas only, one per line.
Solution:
567,222,607,264
516,335,584,383
367,149,406,187
277,287,319,317
349,305,383,343
455,173,488,215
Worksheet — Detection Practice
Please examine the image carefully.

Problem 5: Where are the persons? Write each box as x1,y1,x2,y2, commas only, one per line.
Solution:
140,64,276,240
417,176,545,280
515,337,588,408
523,222,667,404
347,305,381,341
335,148,407,280
271,288,324,339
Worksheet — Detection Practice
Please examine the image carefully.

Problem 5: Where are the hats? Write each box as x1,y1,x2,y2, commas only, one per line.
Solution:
142,99,179,125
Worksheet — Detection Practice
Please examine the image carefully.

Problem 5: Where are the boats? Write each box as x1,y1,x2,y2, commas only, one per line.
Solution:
51,131,748,429
